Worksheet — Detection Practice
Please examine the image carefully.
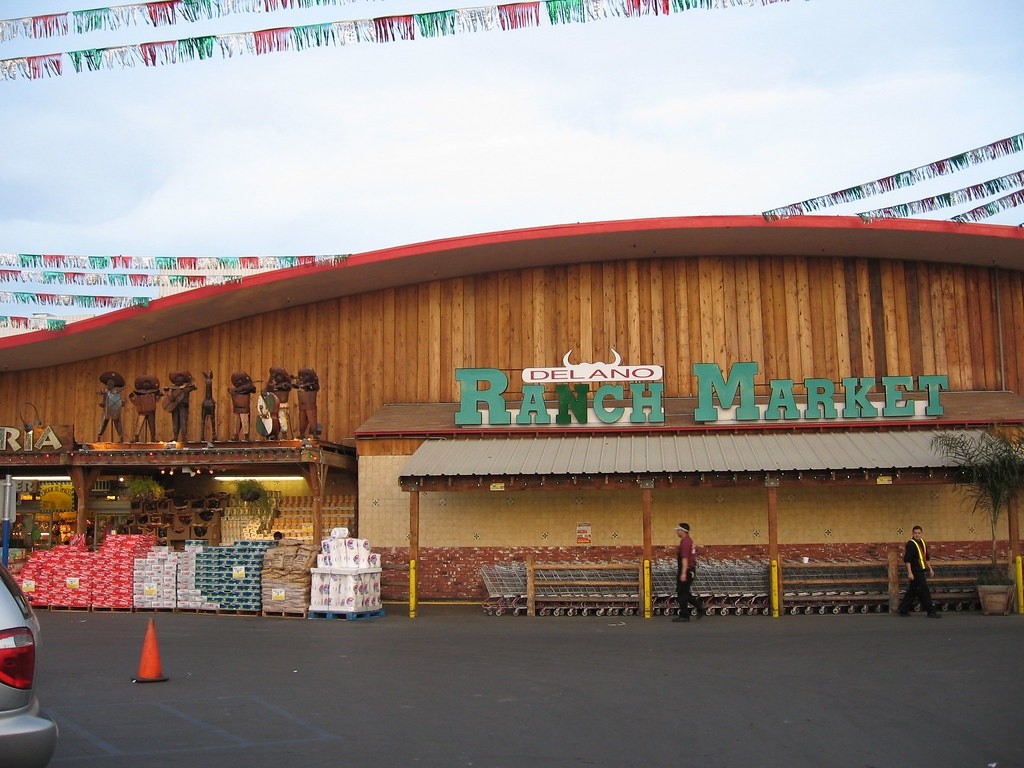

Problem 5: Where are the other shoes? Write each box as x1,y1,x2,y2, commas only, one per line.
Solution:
895,608,911,617
696,606,706,620
672,616,690,622
928,613,941,618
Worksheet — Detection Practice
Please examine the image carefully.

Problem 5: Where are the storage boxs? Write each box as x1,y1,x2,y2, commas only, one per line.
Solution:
7,490,382,612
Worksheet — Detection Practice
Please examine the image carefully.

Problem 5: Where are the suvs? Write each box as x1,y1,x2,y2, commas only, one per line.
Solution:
0,558,59,768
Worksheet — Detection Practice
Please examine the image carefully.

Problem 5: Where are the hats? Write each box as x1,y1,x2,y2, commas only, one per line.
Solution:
674,525,689,532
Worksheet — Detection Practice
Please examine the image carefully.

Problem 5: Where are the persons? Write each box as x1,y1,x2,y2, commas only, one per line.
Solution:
96,371,128,443
896,526,941,618
672,523,707,622
227,371,256,442
291,368,320,440
129,375,164,442
261,367,292,440
162,370,197,444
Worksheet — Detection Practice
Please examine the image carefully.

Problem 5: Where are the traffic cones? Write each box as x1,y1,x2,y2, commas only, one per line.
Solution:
132,620,170,684
